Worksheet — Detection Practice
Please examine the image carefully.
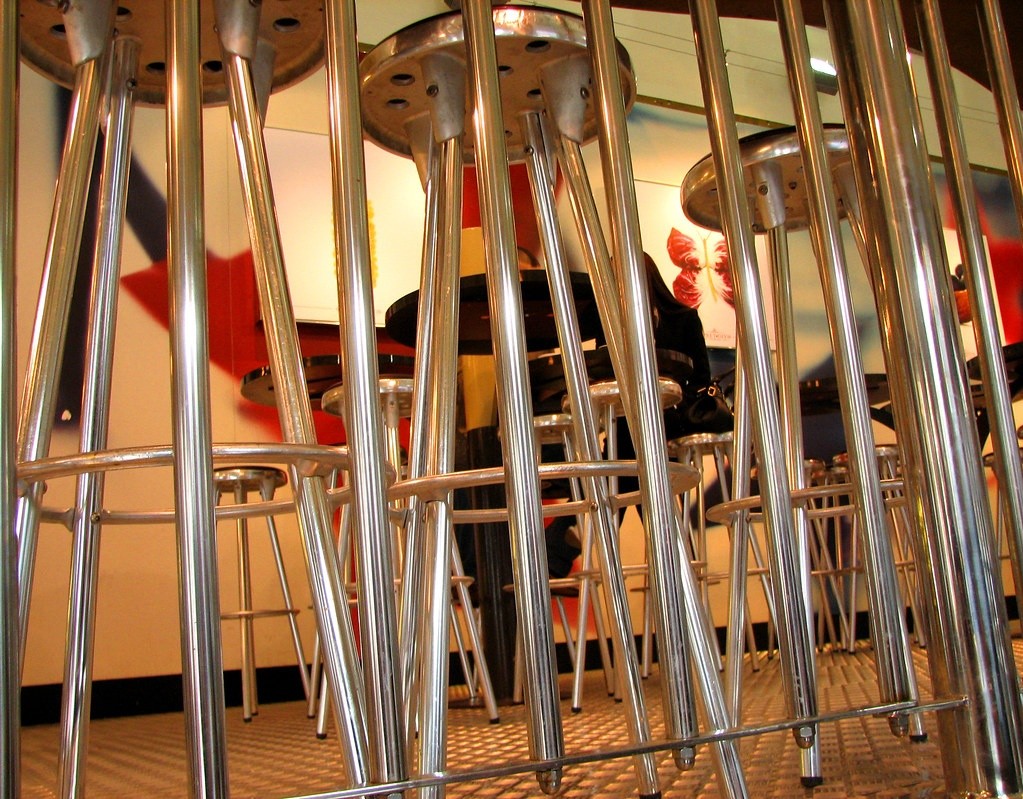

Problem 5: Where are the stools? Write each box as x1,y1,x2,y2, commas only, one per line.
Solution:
0,0,1023,797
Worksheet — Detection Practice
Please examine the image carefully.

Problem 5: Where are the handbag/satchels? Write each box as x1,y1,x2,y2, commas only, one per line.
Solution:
689,386,734,433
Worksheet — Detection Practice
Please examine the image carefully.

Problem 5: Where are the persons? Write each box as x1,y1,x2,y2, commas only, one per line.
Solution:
448,244,584,602
541,251,710,576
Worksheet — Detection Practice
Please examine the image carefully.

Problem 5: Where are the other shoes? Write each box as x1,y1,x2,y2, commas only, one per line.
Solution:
536,528,573,578
